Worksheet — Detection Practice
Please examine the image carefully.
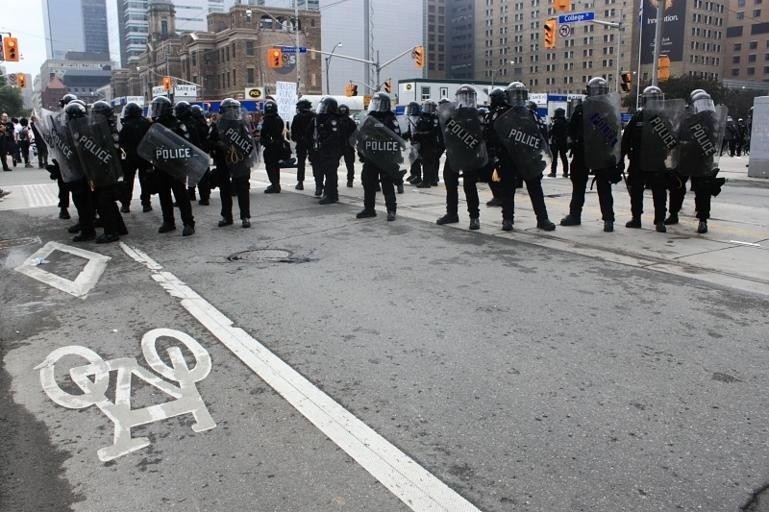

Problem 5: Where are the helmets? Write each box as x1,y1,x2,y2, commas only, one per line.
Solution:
405,82,529,119
372,93,390,112
316,97,338,116
688,90,716,116
58,94,242,127
339,105,350,116
263,101,277,115
642,86,665,111
586,77,610,104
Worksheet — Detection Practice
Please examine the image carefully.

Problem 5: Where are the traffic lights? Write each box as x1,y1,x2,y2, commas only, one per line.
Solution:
544,18,557,50
2,36,19,62
270,48,283,68
415,47,426,68
163,77,171,90
384,80,393,94
621,70,631,92
17,73,26,88
350,83,358,97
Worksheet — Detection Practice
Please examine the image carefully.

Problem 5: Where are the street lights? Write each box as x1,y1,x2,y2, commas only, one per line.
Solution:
246,8,300,97
324,42,343,94
491,59,517,90
90,89,106,98
136,65,205,108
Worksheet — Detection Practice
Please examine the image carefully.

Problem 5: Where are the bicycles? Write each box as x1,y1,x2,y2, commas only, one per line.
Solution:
726,134,750,156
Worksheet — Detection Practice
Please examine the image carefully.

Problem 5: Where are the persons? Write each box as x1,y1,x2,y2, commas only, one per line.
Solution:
1,93,263,245
556,75,623,232
488,80,558,232
263,91,753,223
434,84,484,231
666,89,725,235
620,84,675,233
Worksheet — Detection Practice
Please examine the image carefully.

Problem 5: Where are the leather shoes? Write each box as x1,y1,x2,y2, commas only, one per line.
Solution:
560,213,708,233
398,183,404,193
183,223,195,236
187,189,196,201
537,217,556,231
356,203,376,218
503,219,512,231
143,204,152,212
264,179,353,204
59,205,130,244
218,218,233,227
487,198,502,206
242,218,250,228
407,175,439,188
159,224,176,233
437,212,459,225
387,209,396,220
199,199,210,206
470,217,480,230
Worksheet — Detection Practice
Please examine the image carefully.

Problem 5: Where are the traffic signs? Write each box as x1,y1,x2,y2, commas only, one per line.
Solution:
281,46,307,53
558,11,595,23
